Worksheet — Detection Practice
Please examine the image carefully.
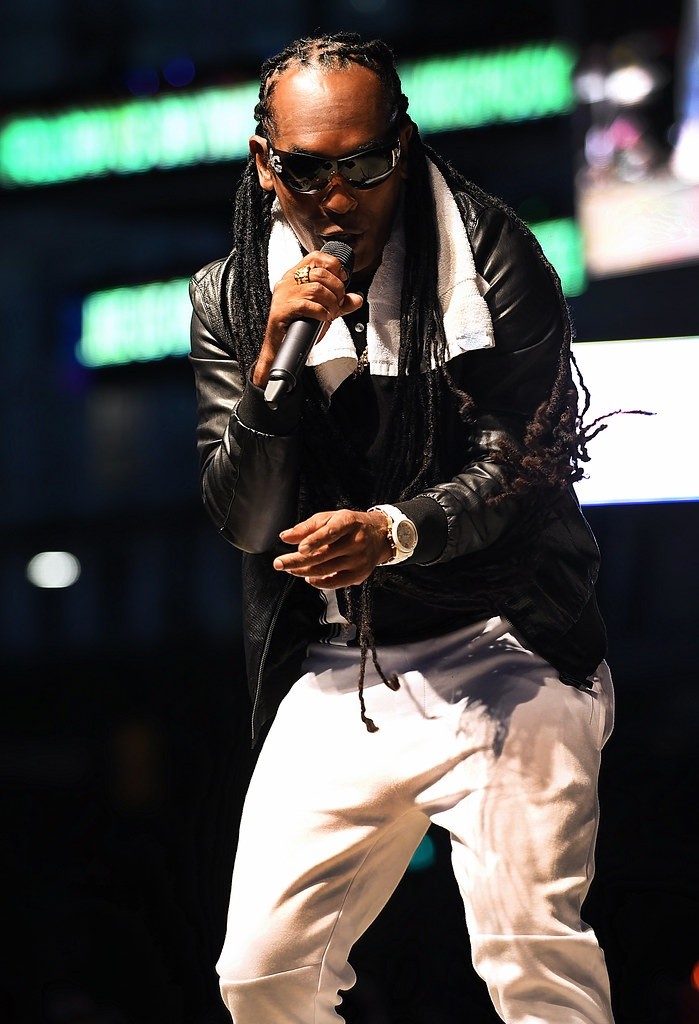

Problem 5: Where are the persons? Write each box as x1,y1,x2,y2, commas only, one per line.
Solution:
188,30,617,1024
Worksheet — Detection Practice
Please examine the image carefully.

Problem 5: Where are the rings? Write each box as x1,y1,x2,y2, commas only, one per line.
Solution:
294,265,311,285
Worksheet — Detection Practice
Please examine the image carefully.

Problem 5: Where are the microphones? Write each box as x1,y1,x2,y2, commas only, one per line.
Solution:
263,240,354,410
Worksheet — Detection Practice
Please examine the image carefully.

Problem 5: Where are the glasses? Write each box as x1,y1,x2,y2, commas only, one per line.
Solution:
266,136,402,195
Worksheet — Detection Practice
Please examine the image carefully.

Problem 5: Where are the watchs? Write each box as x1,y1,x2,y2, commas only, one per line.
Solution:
366,503,419,566
